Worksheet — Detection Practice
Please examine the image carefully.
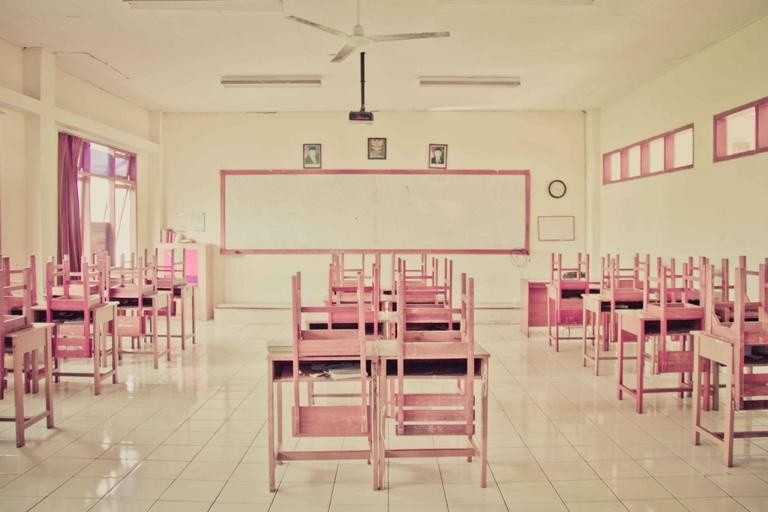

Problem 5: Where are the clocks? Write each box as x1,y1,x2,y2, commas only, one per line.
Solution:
549,180,566,198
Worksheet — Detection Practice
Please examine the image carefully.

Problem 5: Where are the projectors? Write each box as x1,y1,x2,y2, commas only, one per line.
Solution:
349,111,374,121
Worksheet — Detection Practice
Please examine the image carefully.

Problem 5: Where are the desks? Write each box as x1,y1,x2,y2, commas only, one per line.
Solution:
266,339,491,492
690,330,768,466
0,322,57,448
132,284,198,350
520,278,643,338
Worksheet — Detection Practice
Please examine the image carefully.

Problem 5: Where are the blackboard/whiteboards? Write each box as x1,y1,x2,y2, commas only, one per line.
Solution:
220,170,531,255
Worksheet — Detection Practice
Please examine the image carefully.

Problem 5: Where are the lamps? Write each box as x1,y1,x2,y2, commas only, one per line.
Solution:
220,80,326,88
419,76,521,87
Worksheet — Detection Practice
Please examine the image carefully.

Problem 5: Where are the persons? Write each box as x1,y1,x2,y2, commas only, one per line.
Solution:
431,148,444,163
305,146,319,164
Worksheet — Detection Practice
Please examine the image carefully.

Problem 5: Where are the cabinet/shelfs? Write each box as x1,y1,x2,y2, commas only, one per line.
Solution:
152,242,214,322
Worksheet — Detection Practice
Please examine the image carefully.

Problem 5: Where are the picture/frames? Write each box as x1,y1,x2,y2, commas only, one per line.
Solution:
303,144,321,169
368,138,386,159
429,144,448,169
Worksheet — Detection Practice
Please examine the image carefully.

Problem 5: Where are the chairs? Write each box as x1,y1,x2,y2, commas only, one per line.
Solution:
550,252,768,411
290,252,474,437
0,247,188,399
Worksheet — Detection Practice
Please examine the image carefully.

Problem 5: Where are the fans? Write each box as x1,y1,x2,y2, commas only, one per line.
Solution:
283,0,451,64
